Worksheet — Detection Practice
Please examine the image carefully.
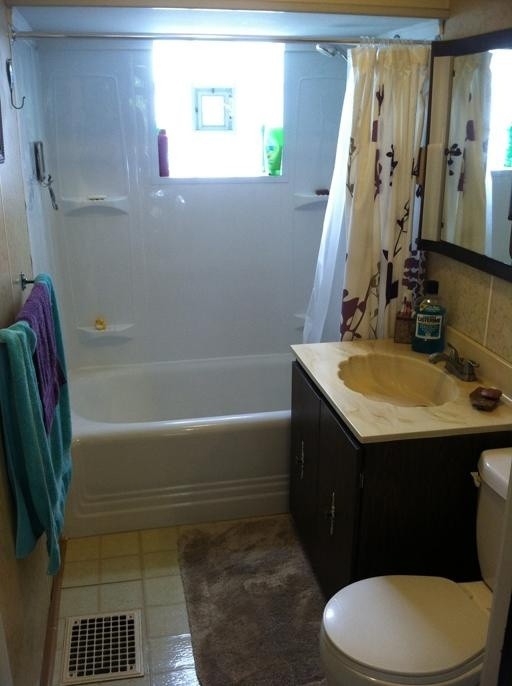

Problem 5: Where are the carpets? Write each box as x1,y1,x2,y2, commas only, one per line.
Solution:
178,514,329,686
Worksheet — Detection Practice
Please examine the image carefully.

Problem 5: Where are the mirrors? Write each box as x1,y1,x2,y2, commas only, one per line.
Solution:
415,27,512,282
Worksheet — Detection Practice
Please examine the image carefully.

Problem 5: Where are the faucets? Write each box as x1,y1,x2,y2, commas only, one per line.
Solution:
428,352,459,368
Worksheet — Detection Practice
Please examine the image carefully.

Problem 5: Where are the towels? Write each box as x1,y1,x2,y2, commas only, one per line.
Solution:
0,273,73,576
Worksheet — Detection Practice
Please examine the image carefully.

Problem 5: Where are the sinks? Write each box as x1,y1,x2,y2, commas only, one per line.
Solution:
338,353,460,408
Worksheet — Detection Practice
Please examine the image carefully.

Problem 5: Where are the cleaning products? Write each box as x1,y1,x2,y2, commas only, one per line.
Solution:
158,128,170,176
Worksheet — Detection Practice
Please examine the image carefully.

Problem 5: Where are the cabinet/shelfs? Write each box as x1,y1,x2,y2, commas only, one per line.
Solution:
289,362,512,602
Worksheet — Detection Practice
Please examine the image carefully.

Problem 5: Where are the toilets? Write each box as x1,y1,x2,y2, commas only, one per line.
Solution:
319,446,512,685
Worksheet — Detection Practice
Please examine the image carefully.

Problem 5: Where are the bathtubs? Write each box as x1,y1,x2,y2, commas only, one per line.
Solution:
57,351,294,537
